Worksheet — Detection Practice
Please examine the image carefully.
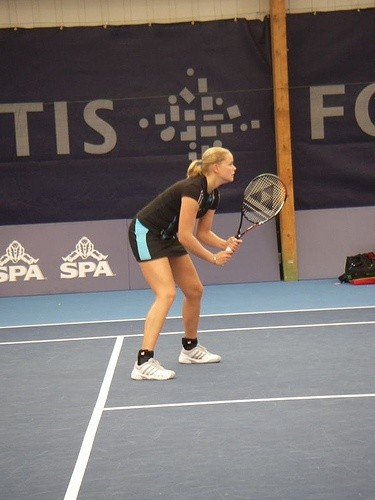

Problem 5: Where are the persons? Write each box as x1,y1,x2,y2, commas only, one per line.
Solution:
126,146,243,383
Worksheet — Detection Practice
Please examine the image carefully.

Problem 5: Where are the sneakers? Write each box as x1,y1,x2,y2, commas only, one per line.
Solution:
131,357,175,381
179,343,221,365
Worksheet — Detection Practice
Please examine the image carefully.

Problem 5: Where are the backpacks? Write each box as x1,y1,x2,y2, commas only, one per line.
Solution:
338,253,375,286
